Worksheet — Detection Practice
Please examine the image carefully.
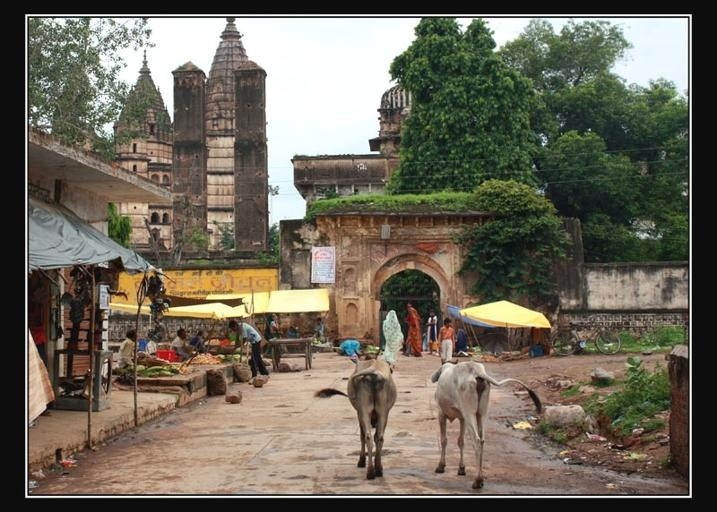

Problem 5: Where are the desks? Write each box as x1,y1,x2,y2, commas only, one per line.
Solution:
270,335,314,372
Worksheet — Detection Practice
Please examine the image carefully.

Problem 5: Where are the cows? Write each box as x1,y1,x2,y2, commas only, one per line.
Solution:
432,358,542,489
314,349,396,480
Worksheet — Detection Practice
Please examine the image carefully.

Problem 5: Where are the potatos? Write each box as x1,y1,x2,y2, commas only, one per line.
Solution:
191,353,221,365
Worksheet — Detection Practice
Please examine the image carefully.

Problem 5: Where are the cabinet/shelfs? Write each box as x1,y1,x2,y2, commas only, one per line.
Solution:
50,348,112,413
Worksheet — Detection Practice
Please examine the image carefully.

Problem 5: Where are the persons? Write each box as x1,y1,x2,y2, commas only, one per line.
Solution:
228,320,272,384
340,339,364,357
437,317,456,364
456,328,467,357
145,318,167,354
286,326,300,352
382,309,405,374
312,317,323,338
425,309,439,357
118,329,136,370
404,303,422,357
263,314,284,357
171,328,205,357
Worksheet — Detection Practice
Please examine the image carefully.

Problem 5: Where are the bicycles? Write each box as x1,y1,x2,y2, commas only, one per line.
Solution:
548,321,621,356
